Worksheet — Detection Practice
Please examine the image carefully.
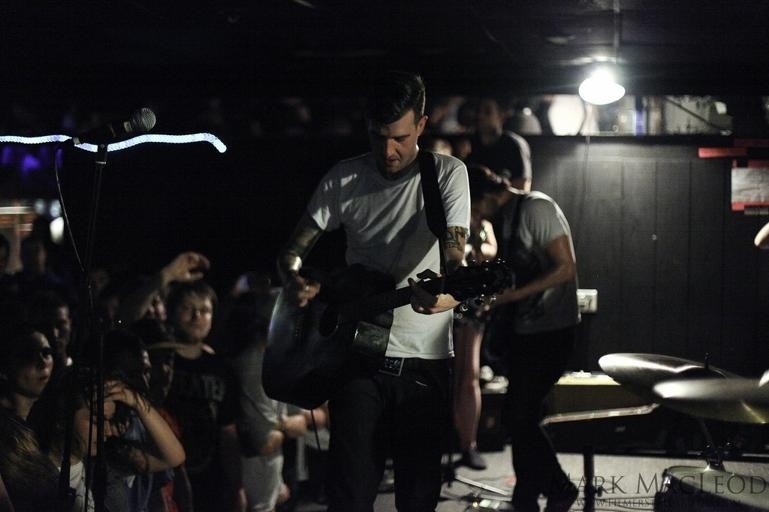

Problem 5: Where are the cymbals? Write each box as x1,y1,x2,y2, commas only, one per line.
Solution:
597,353,743,405
654,378,765,424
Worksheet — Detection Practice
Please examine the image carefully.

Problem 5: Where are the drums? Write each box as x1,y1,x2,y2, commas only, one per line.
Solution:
649,464,769,511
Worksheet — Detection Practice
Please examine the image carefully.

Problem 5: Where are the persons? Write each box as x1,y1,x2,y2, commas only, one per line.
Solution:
1,72,583,511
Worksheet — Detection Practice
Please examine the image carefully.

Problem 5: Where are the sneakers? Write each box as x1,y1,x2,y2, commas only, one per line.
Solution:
545,482,578,512
464,447,486,469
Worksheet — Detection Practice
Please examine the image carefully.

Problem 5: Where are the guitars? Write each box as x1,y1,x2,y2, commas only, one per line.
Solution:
261,259,512,409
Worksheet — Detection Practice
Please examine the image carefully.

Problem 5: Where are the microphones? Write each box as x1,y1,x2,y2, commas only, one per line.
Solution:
58,108,157,149
